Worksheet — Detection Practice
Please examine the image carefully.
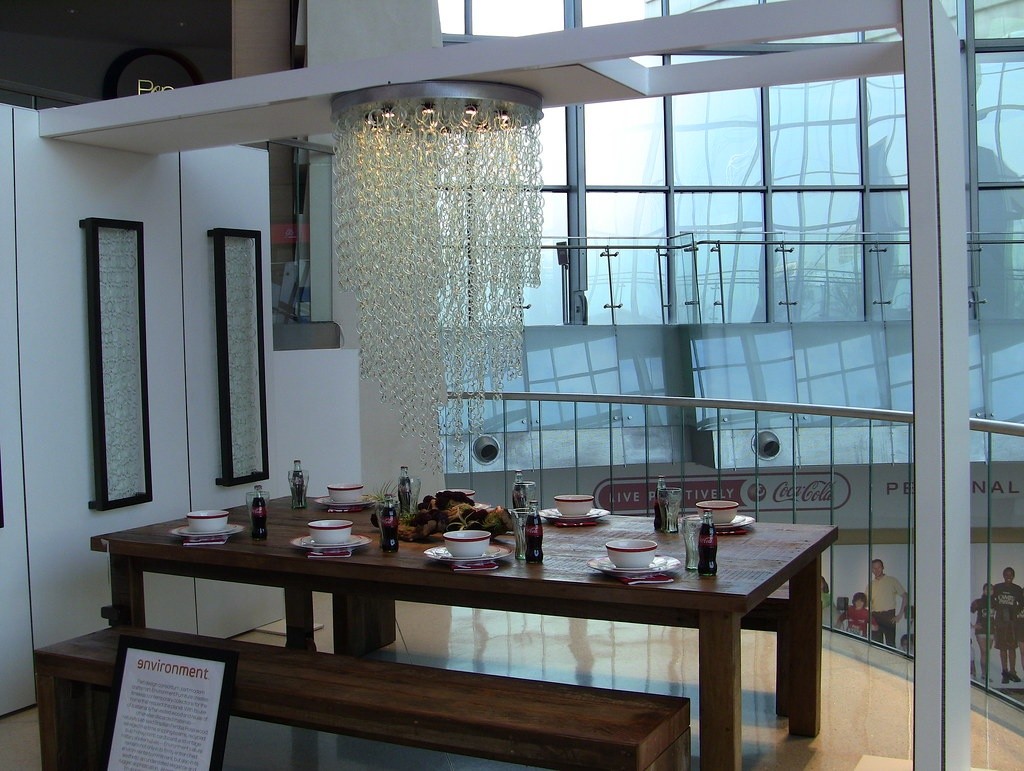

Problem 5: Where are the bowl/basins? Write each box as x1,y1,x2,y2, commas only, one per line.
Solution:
442,531,493,557
696,500,739,522
554,494,657,568
186,510,231,533
308,483,363,545
436,488,475,501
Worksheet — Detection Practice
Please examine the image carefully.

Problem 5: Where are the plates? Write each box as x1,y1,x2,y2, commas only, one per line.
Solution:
423,545,510,561
539,507,680,576
292,495,377,551
169,524,246,538
474,504,492,511
683,516,757,530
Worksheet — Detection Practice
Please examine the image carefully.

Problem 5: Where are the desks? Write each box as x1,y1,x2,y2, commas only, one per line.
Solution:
89,497,839,771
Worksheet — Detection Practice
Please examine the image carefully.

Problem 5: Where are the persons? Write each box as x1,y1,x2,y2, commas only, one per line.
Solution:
834,591,879,637
990,567,1024,684
864,558,908,648
821,577,844,630
971,582,995,681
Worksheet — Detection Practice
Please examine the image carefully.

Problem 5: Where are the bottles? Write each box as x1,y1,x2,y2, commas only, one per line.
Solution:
512,471,525,511
381,495,399,551
654,475,669,530
524,501,544,562
398,466,412,514
698,509,717,576
291,460,305,511
251,484,268,539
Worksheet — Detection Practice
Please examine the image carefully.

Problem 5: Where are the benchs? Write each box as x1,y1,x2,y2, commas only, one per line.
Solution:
33,625,692,771
740,586,791,719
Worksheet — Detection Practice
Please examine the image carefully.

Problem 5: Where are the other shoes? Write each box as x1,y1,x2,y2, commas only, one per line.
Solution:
1008,671,1020,682
1002,671,1009,683
981,674,993,685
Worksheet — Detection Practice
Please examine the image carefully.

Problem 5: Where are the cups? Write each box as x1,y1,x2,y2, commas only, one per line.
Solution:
659,487,682,533
511,507,529,560
512,482,537,509
680,515,700,571
246,492,271,537
288,471,309,508
399,478,421,516
374,500,399,549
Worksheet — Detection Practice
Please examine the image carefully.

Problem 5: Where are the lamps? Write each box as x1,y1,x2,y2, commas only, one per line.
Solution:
330,80,542,474
